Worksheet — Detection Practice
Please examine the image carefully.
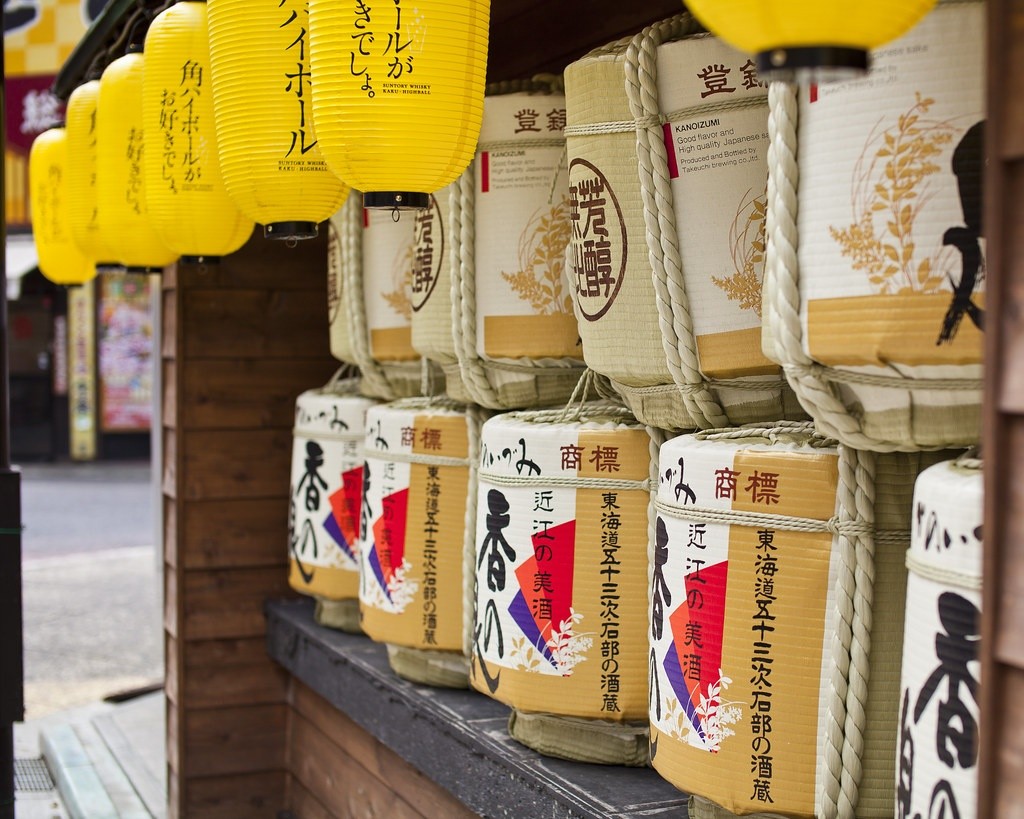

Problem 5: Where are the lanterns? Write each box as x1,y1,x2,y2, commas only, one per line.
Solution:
686,0,937,83
207,0,490,246
29,1,256,291
